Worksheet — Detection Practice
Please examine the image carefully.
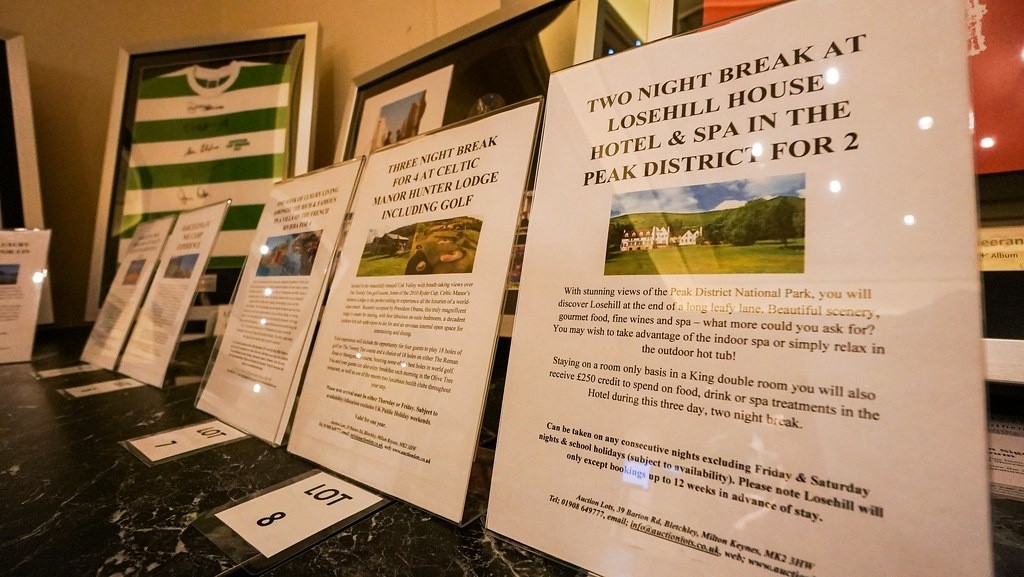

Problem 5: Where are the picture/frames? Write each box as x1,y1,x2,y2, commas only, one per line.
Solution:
339,0,606,334
0,29,55,326
645,0,1024,384
85,20,323,323
